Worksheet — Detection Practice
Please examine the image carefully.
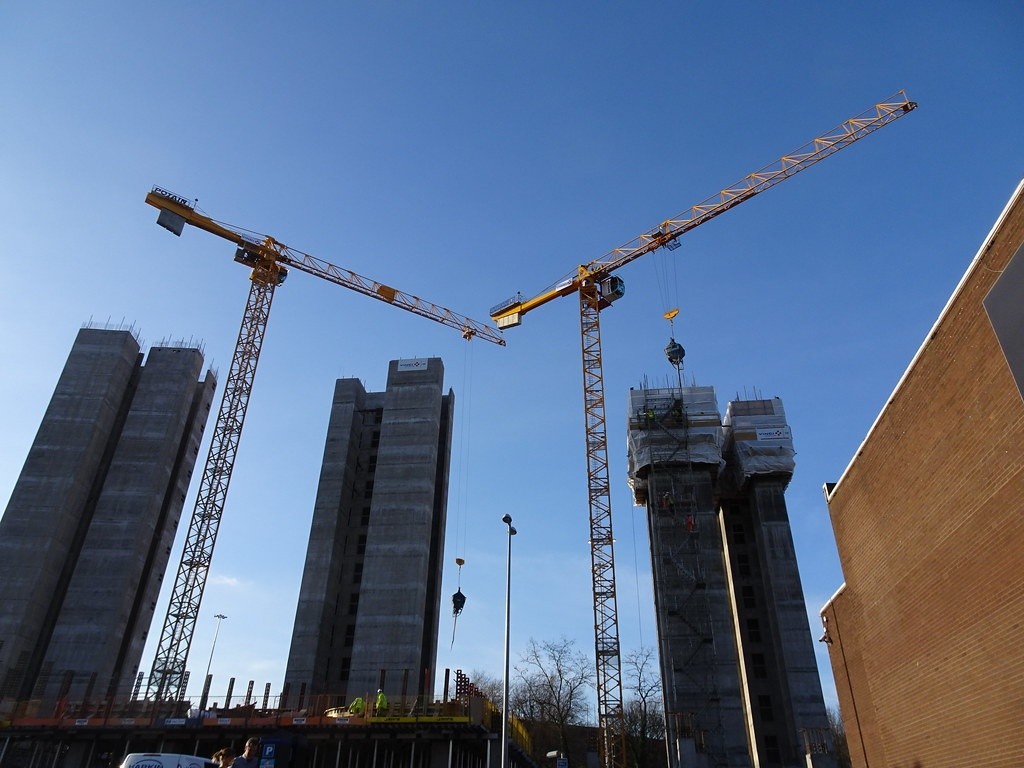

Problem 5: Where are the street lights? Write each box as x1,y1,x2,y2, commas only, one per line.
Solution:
206,613,229,676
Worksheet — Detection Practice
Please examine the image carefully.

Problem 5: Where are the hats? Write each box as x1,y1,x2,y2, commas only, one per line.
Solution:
245,737,258,747
376,689,382,693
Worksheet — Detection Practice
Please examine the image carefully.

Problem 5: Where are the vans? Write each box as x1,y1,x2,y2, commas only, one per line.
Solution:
119,753,222,768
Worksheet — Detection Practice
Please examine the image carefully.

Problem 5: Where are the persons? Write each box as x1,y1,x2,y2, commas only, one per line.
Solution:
376,689,387,716
212,748,235,768
231,737,260,768
348,696,365,718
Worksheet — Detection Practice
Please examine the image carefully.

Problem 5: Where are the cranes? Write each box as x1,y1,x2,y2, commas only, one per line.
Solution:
490,89,919,768
143,185,506,706
